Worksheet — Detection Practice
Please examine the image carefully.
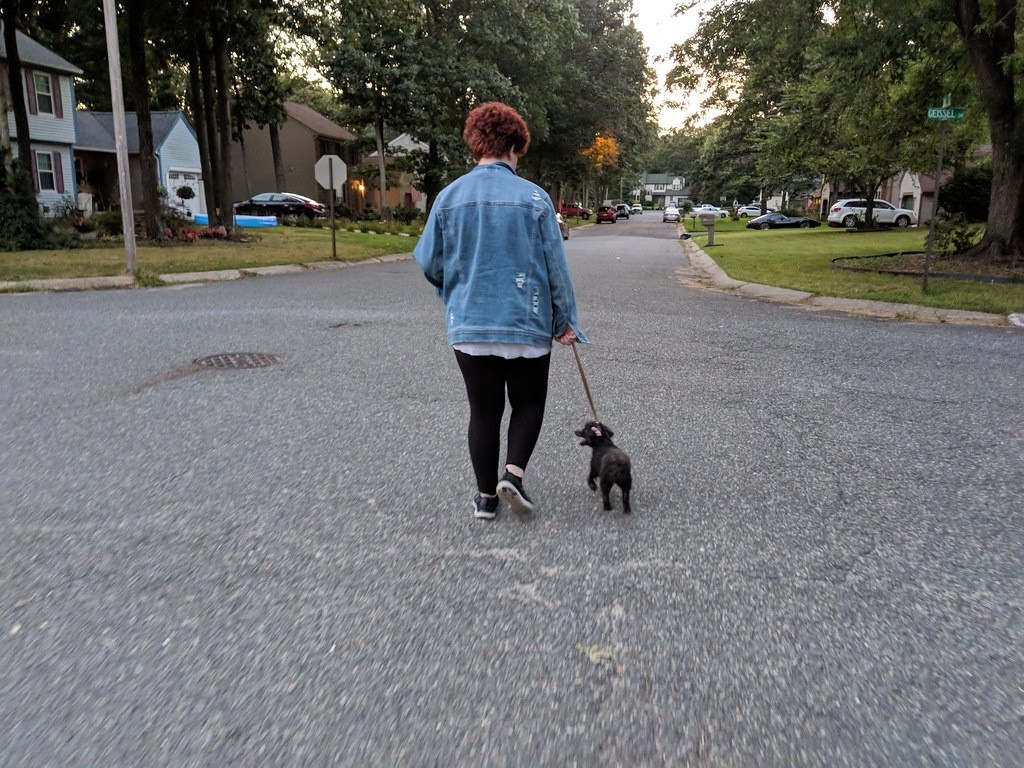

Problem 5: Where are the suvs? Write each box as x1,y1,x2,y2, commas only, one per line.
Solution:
614,203,631,221
826,198,917,229
596,205,616,224
561,202,593,220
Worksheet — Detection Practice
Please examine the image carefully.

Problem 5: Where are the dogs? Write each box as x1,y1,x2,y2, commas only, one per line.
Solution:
574,421,633,516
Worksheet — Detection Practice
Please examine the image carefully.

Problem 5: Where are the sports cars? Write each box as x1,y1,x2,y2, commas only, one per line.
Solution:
746,212,822,230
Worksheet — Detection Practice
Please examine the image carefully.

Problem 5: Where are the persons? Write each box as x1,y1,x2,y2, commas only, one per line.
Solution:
412,101,590,520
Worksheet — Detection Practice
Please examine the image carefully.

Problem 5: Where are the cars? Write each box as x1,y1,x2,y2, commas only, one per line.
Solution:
556,212,570,240
233,191,327,228
631,204,644,215
662,208,681,223
691,207,730,219
737,206,772,218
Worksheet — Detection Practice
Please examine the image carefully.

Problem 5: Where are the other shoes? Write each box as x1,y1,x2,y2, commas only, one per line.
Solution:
496,468,536,519
472,494,500,520
